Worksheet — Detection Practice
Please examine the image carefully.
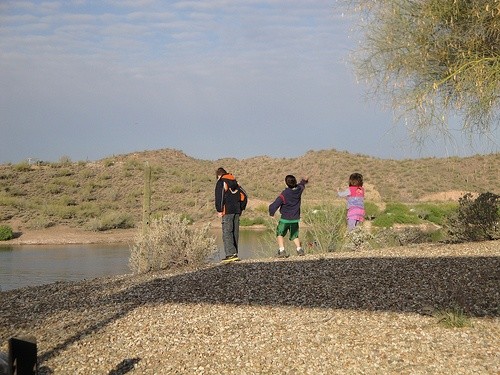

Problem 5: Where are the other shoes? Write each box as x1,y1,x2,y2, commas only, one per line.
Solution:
297,249,305,255
272,250,288,257
221,255,240,262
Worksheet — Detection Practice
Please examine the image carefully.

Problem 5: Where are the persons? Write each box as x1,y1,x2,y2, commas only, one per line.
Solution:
214,168,248,261
269,174,312,257
337,172,365,227
